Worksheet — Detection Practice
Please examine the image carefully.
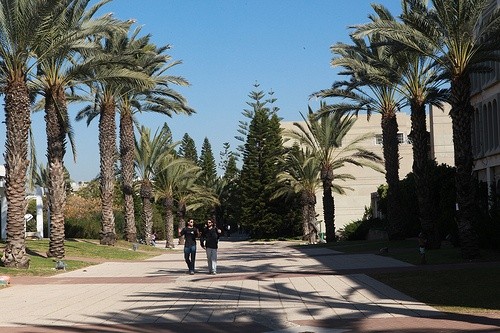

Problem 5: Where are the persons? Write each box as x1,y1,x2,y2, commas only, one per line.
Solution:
178,219,202,275
200,219,219,275
418,234,427,264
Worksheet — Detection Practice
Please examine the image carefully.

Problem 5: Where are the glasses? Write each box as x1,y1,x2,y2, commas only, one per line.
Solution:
189,222,193,223
207,222,211,224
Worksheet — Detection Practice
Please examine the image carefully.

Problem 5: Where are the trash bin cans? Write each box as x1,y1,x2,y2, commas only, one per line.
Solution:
319,232,324,240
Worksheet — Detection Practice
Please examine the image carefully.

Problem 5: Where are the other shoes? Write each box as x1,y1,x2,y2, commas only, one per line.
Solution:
190,270,194,274
213,272,216,275
